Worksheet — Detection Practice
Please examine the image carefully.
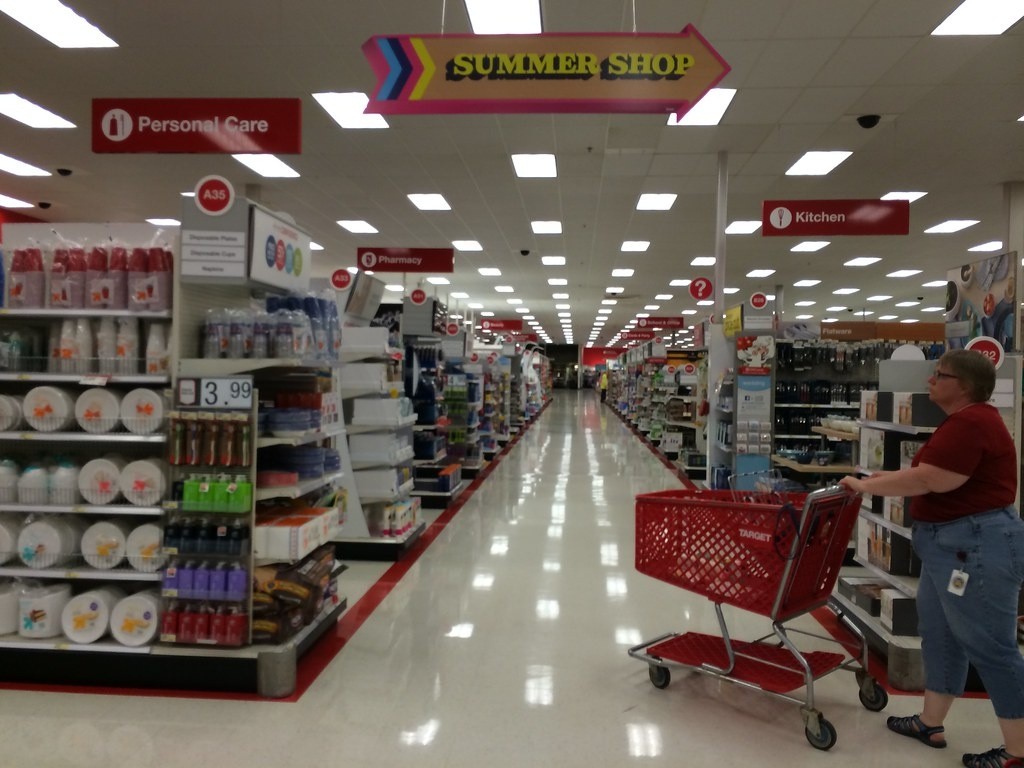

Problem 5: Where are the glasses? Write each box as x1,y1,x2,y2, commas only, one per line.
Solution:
934,369,959,380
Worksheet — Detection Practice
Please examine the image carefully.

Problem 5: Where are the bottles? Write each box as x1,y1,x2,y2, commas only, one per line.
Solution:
202,308,308,358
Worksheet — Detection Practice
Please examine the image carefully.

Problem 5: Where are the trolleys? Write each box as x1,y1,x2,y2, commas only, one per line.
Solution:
628,480,890,751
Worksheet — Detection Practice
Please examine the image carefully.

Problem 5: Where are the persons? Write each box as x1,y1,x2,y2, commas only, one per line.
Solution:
837,350,1024,768
601,372,607,403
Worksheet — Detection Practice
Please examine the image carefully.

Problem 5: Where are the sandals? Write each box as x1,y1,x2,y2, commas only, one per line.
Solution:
963,745,1024,768
887,714,948,748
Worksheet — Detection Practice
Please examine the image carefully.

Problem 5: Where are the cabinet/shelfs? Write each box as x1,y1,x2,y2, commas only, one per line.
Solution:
334,276,555,562
605,303,945,691
0,196,348,699
830,352,1024,690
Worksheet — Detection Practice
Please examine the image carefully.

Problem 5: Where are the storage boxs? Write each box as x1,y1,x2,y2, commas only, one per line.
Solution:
857,474,881,513
838,575,884,618
881,495,915,528
855,425,898,472
898,437,927,470
686,451,706,467
892,391,950,427
879,588,919,636
861,522,922,575
255,506,339,561
858,390,893,421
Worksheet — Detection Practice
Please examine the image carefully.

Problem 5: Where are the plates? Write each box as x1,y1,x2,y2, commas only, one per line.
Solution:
0,386,170,647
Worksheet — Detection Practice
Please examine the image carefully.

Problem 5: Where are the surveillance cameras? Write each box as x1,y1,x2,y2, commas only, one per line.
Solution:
848,309,853,312
37,202,52,209
57,168,72,176
520,250,530,256
611,293,617,295
856,115,882,128
917,296,923,300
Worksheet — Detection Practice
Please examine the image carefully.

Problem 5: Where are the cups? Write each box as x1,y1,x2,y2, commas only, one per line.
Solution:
8,247,170,309
43,318,171,374
